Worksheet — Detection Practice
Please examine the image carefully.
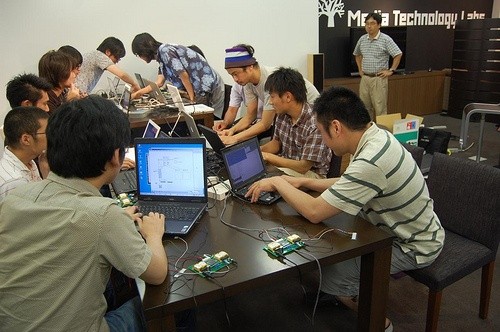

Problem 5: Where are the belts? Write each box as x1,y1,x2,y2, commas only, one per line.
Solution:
363,73,381,77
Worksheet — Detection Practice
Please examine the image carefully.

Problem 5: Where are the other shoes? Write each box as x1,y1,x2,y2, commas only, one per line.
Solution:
385,321,394,332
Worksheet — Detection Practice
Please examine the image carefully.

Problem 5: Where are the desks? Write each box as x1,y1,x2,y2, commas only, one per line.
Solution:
107,137,390,332
107,90,213,136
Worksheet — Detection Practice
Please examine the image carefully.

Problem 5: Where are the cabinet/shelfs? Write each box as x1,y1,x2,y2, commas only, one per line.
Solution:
326,70,446,114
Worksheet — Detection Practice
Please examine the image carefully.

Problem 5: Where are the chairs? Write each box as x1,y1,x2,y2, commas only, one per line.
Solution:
402,152,500,332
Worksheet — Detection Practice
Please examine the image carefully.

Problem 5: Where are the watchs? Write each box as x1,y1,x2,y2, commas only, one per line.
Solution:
389,68,395,73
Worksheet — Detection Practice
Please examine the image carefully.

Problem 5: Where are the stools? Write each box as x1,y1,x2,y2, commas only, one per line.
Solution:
462,103,500,162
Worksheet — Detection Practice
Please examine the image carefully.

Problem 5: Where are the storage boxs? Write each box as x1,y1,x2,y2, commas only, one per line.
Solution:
376,112,422,147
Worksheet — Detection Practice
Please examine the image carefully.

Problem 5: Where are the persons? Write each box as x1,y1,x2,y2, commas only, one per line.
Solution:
354,13,403,122
0,44,89,203
261,66,333,183
76,36,141,96
0,94,168,332
246,86,446,331
212,79,274,138
218,44,321,149
185,44,225,125
127,31,219,108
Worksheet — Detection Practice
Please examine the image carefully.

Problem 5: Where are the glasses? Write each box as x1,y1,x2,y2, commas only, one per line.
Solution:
113,55,121,63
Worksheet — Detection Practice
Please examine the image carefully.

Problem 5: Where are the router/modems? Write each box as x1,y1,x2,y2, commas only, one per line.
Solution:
207,179,234,201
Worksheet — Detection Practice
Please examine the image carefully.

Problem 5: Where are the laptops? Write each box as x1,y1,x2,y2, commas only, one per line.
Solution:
106,77,118,101
220,135,290,204
135,72,149,96
122,120,162,161
166,84,215,115
180,110,213,149
417,126,451,156
128,137,208,235
198,124,226,161
102,77,120,96
121,89,130,117
112,132,170,195
143,78,193,106
